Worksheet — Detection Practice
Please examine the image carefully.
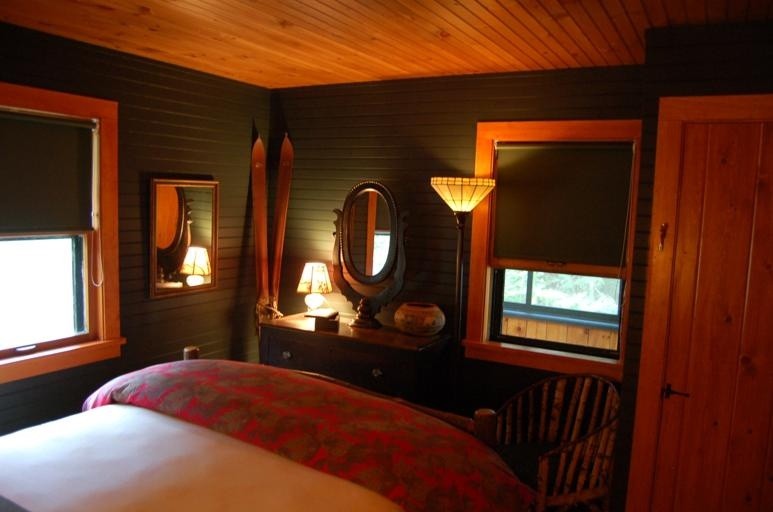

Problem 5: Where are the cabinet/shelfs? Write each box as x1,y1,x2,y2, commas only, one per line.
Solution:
251,310,456,411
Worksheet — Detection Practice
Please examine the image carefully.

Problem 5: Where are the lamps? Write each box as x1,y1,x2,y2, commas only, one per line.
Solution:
295,261,334,313
427,172,501,416
179,245,212,288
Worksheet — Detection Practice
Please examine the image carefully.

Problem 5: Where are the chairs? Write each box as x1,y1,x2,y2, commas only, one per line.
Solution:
472,369,624,512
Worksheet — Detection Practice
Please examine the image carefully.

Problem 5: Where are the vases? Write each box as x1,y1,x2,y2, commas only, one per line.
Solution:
394,299,445,338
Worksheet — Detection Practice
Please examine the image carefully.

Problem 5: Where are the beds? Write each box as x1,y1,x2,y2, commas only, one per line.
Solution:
2,343,499,512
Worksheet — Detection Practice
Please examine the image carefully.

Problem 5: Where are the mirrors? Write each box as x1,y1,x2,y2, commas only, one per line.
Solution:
331,179,417,330
150,177,222,302
157,184,194,276
